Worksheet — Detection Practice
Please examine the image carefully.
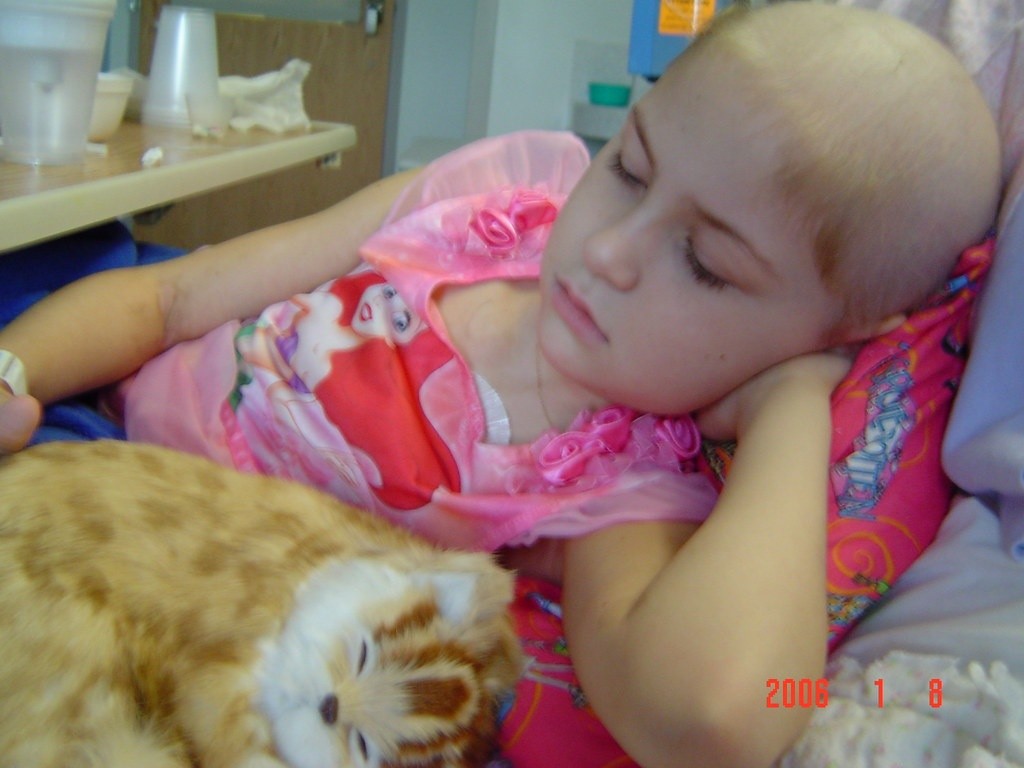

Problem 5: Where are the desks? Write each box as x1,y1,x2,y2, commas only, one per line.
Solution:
0,118,358,253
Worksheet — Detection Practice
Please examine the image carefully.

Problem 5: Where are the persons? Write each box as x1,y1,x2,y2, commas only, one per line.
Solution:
2,1,1006,768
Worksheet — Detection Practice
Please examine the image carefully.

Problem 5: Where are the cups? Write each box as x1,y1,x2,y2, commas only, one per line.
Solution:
1,1,120,166
140,3,223,130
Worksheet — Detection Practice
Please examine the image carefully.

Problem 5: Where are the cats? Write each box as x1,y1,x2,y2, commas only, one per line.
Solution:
1,436,522,768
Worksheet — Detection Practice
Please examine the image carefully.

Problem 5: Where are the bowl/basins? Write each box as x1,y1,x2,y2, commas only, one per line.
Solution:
85,74,137,141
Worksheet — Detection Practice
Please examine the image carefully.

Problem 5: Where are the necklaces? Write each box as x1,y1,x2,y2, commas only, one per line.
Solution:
535,334,556,430
